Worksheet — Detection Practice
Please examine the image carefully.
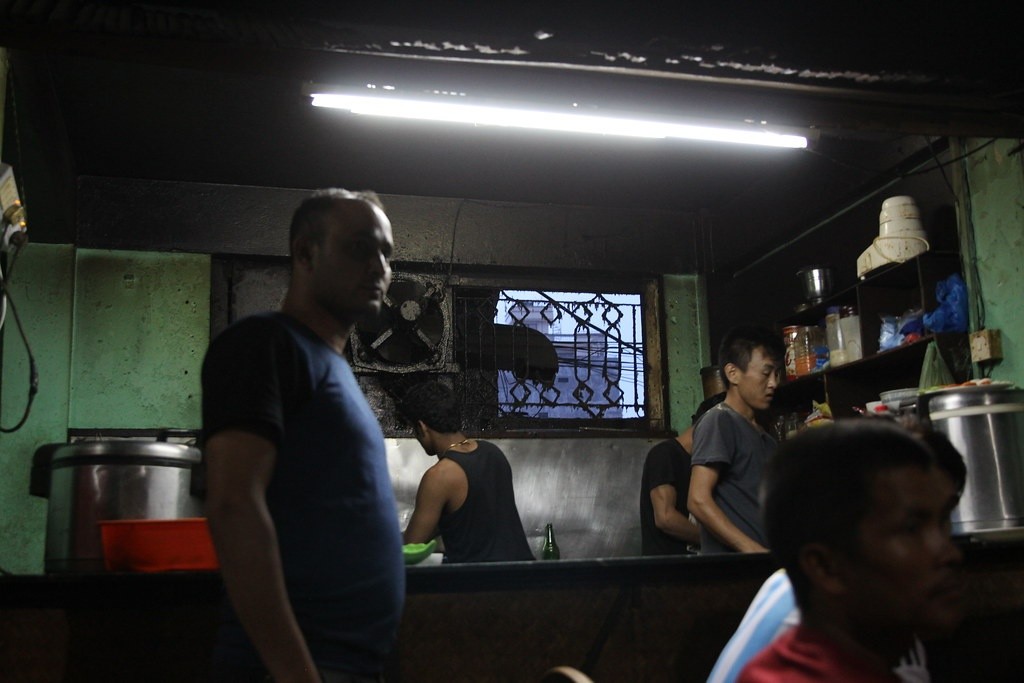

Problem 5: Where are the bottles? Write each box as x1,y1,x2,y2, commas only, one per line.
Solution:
825,306,849,369
794,325,818,377
542,523,560,560
782,325,799,383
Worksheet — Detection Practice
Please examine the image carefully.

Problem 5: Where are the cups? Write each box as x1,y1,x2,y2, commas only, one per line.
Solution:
807,269,826,306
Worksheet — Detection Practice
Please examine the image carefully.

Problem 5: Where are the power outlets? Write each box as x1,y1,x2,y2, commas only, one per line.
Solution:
0,161,28,254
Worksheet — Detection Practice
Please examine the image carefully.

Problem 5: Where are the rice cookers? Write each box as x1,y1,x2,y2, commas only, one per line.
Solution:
32,440,203,575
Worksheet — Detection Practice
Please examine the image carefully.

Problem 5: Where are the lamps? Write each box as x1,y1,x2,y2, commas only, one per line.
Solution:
301,80,821,154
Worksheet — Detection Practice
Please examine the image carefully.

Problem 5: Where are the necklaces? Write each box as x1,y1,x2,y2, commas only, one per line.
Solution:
447,438,468,452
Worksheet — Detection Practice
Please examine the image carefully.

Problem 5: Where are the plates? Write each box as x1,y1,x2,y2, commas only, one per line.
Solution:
944,382,1014,390
969,527,1024,544
402,539,437,565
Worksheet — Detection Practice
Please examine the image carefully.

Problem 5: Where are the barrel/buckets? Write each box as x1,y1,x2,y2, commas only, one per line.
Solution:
928,389,1024,537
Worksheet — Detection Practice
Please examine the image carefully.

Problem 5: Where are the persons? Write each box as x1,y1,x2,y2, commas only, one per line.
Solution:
705,419,967,683
201,189,405,683
688,331,782,553
640,392,728,555
400,382,536,564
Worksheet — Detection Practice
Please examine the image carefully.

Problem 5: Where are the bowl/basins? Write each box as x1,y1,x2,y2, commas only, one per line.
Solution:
865,401,897,417
97,517,219,571
879,387,919,406
